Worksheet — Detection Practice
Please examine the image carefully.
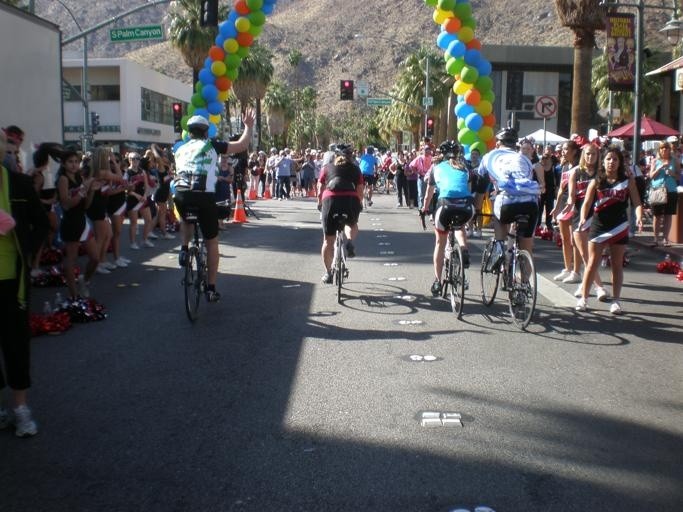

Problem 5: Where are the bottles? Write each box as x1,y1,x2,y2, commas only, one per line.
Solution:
42,301,51,323
602,249,607,267
666,254,670,261
53,293,61,312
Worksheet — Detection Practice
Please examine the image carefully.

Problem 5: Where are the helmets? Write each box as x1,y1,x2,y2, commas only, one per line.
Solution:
335,143,353,155
495,127,518,140
440,140,459,152
366,145,373,151
187,115,210,127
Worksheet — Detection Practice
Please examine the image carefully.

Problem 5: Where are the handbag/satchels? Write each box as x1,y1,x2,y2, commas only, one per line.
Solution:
634,165,645,197
648,174,668,206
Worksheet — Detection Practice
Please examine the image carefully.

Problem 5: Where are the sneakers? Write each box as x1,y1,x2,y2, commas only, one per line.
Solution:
574,284,582,297
473,231,482,237
103,262,118,269
346,241,355,258
431,282,441,296
610,302,621,314
575,298,586,311
179,251,187,265
553,269,570,280
147,232,158,238
130,243,139,250
14,405,37,438
597,288,610,301
562,271,582,284
490,253,504,272
208,291,220,302
97,267,109,273
462,250,470,268
114,260,128,267
136,226,139,234
322,274,333,284
161,232,175,239
122,257,132,264
466,231,473,238
143,241,154,247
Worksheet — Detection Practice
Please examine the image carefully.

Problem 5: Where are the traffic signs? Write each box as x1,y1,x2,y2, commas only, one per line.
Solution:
109,24,164,42
423,97,434,106
357,80,369,97
367,98,391,105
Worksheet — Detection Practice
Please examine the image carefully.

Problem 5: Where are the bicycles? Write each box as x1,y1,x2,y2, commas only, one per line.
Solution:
418,209,479,319
376,170,386,194
364,176,369,208
479,213,537,330
181,212,219,321
329,212,349,304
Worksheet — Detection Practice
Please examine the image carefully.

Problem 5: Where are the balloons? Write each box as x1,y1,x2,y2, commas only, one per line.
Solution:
170,181,175,193
174,0,276,150
424,0,497,153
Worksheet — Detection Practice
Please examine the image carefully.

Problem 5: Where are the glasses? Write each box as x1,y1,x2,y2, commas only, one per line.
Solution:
6,150,20,154
541,156,548,161
661,147,668,149
111,160,119,164
130,157,141,160
623,155,631,159
19,136,24,142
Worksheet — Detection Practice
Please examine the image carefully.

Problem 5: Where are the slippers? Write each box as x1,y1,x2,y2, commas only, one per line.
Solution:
663,242,671,247
649,242,658,247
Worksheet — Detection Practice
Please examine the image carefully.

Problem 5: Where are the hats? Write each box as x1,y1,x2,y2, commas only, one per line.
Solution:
667,136,678,143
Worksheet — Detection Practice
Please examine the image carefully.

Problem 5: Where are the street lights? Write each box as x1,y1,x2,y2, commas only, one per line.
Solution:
354,33,364,39
533,95,558,151
600,0,683,237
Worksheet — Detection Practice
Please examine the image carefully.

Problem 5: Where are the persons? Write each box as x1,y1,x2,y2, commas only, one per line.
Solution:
26,151,57,279
520,142,546,196
374,148,418,209
409,145,432,216
88,151,95,178
124,154,154,250
317,144,365,284
81,155,90,182
575,148,643,314
121,158,139,235
536,143,543,157
246,147,323,199
110,153,136,267
359,146,378,207
562,143,607,300
555,145,562,199
467,148,488,238
436,148,443,160
621,150,642,238
639,150,646,176
56,152,101,300
322,144,337,167
424,138,436,153
353,149,358,161
458,146,466,161
175,107,256,302
475,127,533,298
5,126,24,146
141,150,159,239
88,147,122,275
231,150,248,202
676,136,683,186
147,144,176,240
649,141,681,247
666,136,680,186
646,149,655,167
546,144,554,156
216,153,234,230
535,153,556,241
549,140,581,283
0,130,37,439
421,140,476,296
4,140,49,303
48,143,90,271
575,134,588,146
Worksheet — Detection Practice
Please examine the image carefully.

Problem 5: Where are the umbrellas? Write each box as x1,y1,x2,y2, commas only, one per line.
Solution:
516,129,569,147
604,113,680,137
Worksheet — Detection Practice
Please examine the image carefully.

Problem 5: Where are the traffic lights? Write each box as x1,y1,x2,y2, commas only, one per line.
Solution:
340,80,353,100
172,102,183,134
91,111,100,134
427,117,434,136
